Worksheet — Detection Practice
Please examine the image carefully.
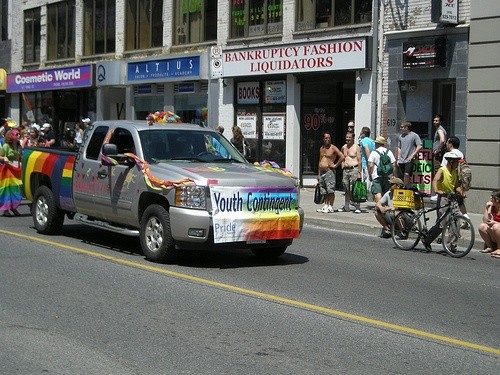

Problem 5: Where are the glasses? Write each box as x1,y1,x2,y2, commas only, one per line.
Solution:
347,126,354,129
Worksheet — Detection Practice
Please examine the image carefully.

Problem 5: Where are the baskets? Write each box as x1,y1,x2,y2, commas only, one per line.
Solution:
392,189,422,209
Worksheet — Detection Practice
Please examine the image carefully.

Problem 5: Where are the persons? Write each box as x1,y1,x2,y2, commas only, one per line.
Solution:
375,178,409,239
368,136,396,213
0,129,23,217
397,120,422,188
440,136,471,228
478,190,500,258
216,126,224,134
316,132,345,213
422,151,466,252
337,132,362,213
429,112,447,202
359,127,375,201
0,117,90,157
230,126,249,158
347,120,355,133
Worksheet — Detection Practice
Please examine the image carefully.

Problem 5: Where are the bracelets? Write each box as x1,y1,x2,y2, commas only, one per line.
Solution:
397,158,401,160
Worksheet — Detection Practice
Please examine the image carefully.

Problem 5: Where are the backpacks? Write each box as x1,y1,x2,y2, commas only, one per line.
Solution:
374,148,393,176
314,183,325,204
451,152,472,191
351,179,368,203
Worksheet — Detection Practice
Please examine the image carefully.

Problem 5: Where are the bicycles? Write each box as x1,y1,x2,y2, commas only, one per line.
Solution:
392,192,475,258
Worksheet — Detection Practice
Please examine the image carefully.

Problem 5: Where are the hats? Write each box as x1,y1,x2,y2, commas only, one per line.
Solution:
82,118,91,125
388,178,404,186
373,136,386,144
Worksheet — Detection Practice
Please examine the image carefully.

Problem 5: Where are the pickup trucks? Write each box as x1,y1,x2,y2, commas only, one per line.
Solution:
22,119,303,263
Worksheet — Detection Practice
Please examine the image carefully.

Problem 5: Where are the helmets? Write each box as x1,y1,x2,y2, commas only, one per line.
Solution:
444,152,462,158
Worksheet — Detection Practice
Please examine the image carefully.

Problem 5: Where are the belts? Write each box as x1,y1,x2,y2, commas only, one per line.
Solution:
344,165,358,169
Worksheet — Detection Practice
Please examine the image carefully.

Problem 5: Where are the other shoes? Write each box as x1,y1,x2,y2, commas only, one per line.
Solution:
316,208,325,212
383,229,391,238
3,211,11,216
355,206,361,212
430,195,438,201
421,238,432,252
338,207,350,211
401,232,409,239
10,208,20,215
481,248,492,253
460,222,468,228
322,209,334,213
491,248,500,254
